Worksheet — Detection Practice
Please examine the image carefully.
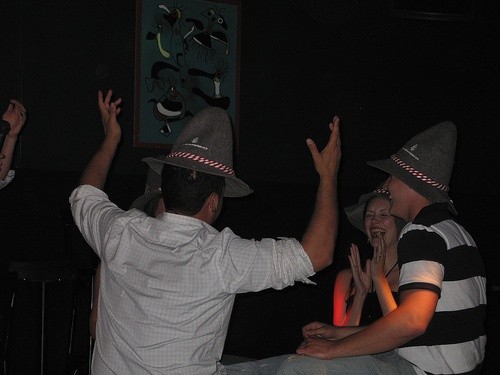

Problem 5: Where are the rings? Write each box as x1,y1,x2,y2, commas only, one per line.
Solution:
355,266,358,267
378,255,382,258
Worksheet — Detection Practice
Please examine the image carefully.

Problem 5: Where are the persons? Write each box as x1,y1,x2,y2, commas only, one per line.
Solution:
334,179,400,327
89,168,165,341
0,100,26,189
69,90,341,375
296,122,487,375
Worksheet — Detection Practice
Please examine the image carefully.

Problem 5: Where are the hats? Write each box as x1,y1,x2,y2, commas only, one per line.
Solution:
341,181,408,234
141,106,254,198
366,121,458,216
141,154,164,199
